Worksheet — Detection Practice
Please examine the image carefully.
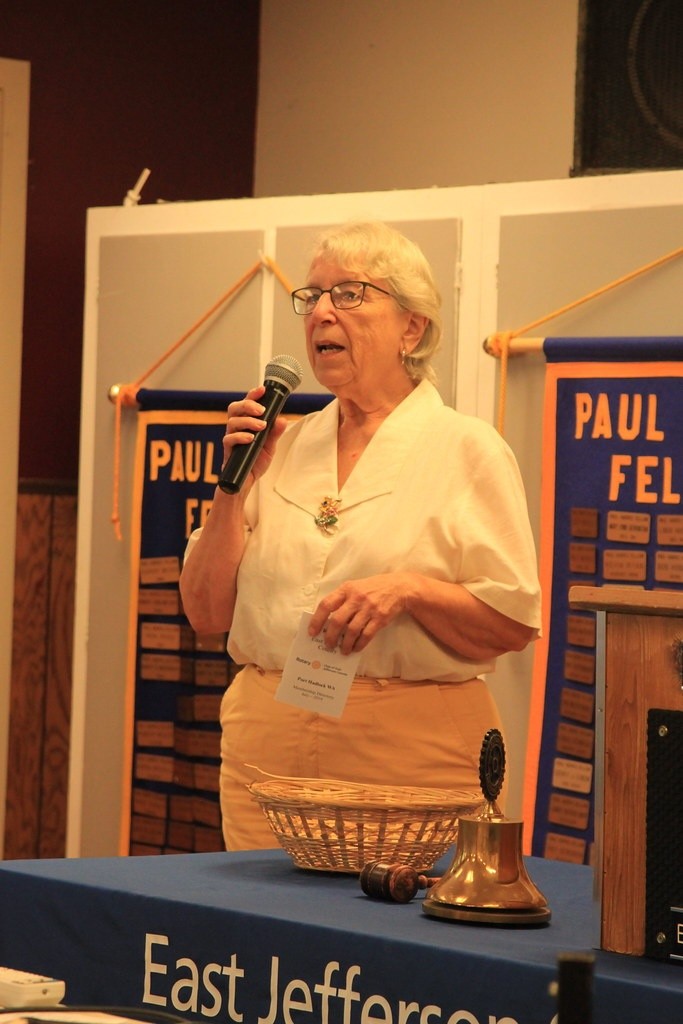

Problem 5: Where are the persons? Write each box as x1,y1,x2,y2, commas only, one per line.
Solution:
179,221,542,853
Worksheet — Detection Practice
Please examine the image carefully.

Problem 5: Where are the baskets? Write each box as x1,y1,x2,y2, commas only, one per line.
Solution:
245,775,487,874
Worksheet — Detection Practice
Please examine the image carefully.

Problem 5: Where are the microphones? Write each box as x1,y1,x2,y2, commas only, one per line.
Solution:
217,355,304,493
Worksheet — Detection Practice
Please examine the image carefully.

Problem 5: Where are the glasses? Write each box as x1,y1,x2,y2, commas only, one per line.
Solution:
291,281,396,315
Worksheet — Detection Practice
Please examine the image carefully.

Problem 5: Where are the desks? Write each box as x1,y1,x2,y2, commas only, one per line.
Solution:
0,839,683,1024
